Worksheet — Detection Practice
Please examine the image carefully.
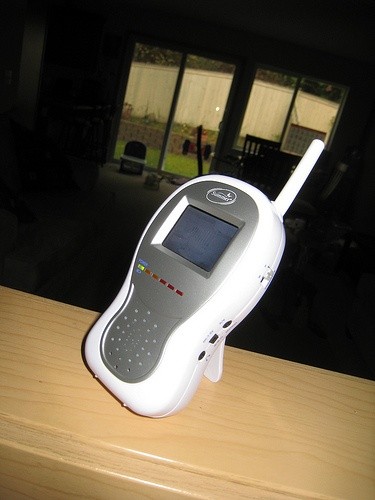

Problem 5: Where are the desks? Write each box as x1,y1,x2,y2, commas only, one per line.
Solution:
263,155,320,195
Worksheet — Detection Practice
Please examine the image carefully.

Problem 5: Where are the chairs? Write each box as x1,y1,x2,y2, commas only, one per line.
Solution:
119,140,146,175
292,160,350,217
236,134,282,187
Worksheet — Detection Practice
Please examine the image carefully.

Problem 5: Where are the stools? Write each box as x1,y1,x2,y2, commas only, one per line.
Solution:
144,173,161,191
